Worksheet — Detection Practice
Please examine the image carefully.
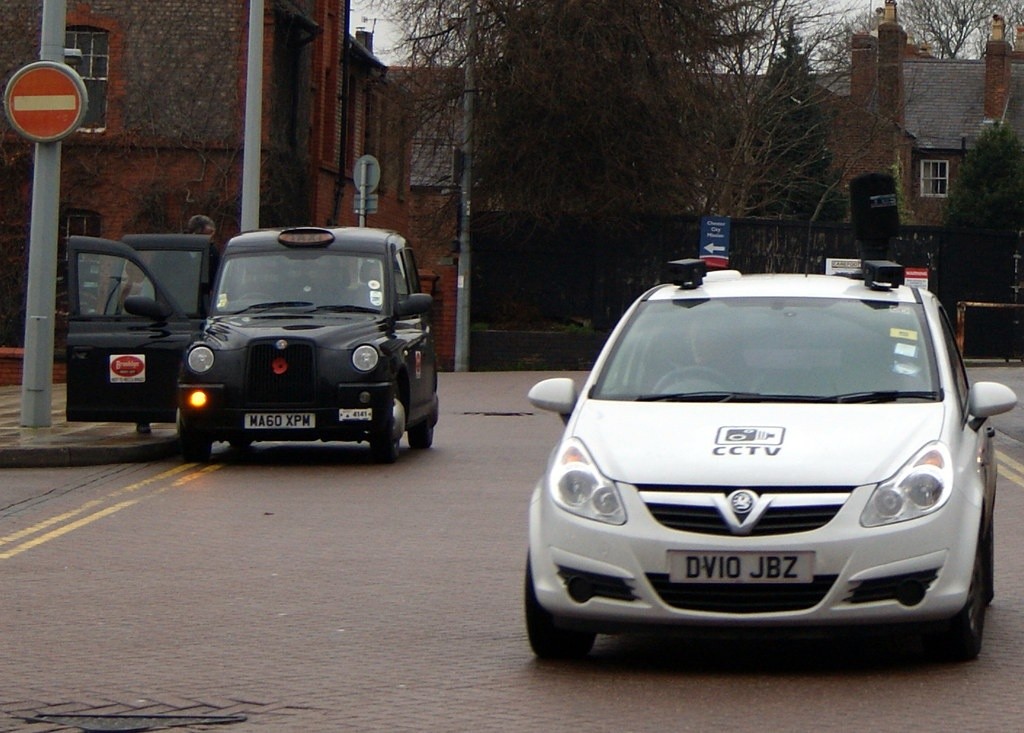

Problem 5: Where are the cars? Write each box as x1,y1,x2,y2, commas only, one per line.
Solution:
65,226,439,464
523,260,1017,665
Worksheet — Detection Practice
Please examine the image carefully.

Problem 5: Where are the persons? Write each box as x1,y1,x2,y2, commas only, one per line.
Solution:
135,215,221,433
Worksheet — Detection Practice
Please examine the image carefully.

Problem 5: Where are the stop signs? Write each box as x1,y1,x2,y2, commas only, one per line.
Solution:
4,61,89,142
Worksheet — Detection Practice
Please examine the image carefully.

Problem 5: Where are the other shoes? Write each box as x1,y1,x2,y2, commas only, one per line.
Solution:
137,425,151,433
